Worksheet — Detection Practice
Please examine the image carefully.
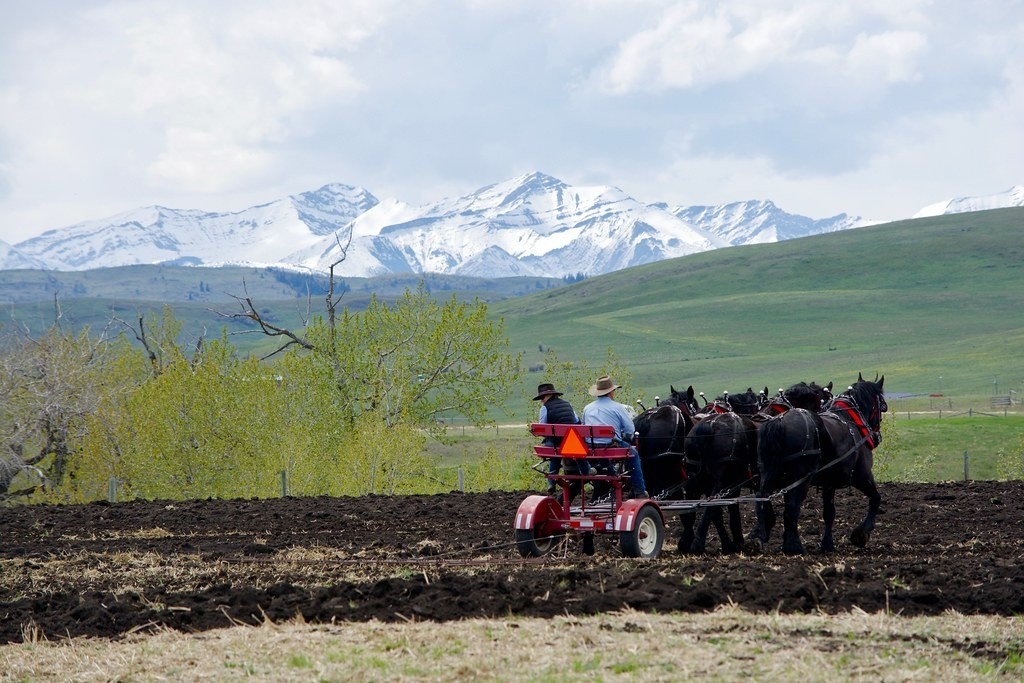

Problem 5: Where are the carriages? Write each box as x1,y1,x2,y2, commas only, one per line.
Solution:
512,370,888,559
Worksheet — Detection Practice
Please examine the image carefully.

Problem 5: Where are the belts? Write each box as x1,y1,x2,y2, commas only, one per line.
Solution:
589,443,612,448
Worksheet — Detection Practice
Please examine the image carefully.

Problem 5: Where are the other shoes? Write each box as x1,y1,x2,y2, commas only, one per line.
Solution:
548,485,556,492
639,491,649,499
558,490,571,506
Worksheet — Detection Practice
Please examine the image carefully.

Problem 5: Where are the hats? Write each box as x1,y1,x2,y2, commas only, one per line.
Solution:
589,375,623,396
532,384,563,400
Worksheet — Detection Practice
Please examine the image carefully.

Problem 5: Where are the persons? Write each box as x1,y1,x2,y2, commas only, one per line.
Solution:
581,375,650,499
537,383,591,497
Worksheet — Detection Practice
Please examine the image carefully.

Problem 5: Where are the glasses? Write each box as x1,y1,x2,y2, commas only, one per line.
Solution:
540,394,549,400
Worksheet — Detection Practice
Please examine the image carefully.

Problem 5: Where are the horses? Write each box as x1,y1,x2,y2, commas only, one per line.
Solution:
581,372,888,560
